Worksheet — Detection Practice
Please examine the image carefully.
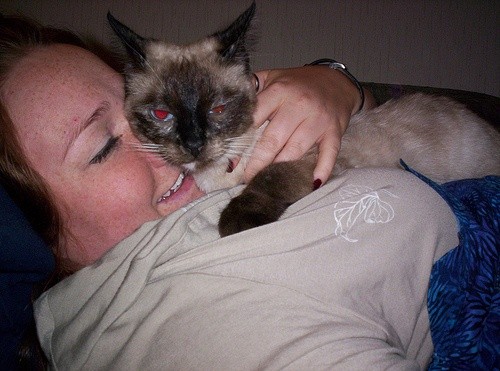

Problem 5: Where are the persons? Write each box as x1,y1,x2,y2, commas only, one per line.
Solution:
1,8,499,371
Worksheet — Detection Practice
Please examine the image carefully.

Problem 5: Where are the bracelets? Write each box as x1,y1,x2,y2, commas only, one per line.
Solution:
302,57,366,117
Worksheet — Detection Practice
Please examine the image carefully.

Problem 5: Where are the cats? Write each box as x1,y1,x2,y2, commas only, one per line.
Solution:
106,0,500,240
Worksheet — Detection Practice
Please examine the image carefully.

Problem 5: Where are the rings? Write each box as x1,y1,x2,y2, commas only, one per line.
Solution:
252,72,261,93
